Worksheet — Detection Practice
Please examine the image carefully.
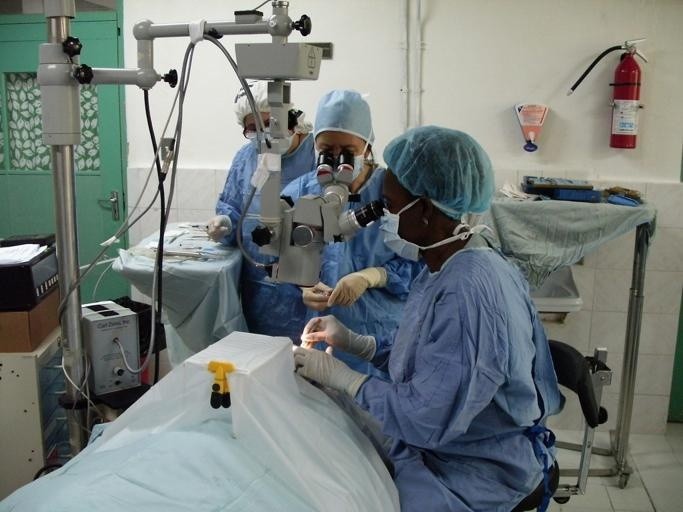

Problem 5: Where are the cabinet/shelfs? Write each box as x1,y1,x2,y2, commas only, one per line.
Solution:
483,191,656,488
0,323,72,500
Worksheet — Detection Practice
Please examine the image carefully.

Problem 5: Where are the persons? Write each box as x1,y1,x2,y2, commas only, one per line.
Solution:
279,88,427,380
293,124,558,512
207,80,316,348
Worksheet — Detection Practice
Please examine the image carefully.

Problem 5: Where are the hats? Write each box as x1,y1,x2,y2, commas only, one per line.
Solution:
311,87,375,146
232,78,306,132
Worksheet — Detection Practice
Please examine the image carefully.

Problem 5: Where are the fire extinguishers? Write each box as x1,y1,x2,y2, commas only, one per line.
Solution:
610,38,648,148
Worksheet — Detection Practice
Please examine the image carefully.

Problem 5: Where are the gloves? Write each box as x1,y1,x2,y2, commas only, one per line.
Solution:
326,264,388,308
298,279,333,312
292,343,372,399
300,313,377,363
206,213,233,243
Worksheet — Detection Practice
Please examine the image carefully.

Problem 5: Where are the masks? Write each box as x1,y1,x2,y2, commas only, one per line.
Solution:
248,112,298,158
313,142,368,184
378,197,447,262
